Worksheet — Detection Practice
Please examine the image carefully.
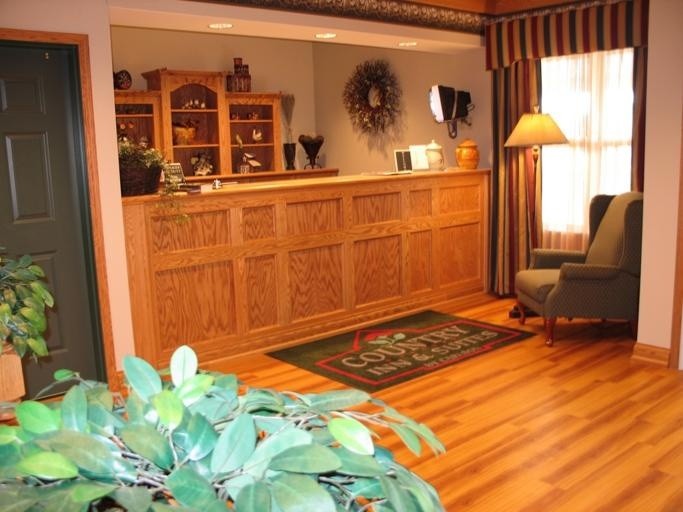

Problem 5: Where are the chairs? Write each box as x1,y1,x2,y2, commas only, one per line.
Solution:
507,189,647,351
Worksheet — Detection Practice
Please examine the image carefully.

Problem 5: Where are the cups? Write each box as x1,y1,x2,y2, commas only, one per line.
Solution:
231,112,240,121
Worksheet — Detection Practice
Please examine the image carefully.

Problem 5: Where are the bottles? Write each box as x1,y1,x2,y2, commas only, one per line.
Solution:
226,58,250,93
239,156,249,175
425,139,445,172
455,138,479,170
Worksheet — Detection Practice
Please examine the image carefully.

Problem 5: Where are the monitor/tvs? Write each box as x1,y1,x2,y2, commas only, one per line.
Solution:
425,85,471,124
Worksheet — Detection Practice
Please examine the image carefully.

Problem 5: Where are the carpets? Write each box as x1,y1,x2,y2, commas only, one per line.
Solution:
256,304,546,400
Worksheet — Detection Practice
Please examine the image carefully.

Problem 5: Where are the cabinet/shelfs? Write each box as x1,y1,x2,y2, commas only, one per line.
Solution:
110,66,339,188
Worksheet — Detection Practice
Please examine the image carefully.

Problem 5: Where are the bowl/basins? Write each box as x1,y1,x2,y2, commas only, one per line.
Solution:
172,125,195,146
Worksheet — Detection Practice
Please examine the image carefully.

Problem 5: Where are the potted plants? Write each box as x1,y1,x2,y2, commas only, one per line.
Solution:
112,129,193,231
0,242,55,427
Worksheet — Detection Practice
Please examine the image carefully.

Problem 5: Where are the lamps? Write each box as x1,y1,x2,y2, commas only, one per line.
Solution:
501,103,570,319
426,82,473,140
